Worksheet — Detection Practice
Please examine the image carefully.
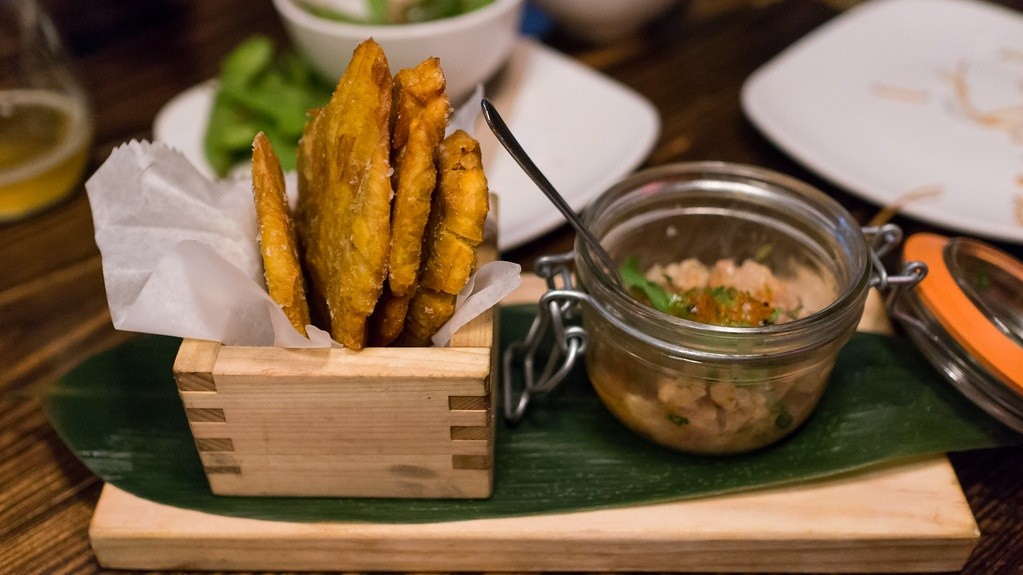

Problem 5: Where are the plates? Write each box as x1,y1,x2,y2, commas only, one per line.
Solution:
736,0,1023,246
150,35,662,255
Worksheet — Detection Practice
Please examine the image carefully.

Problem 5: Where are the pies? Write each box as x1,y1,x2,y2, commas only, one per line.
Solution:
251,39,488,350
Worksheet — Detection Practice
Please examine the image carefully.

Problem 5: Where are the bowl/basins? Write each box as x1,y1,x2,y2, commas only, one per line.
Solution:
272,0,524,106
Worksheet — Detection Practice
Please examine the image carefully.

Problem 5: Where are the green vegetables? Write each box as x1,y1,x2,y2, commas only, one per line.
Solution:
288,0,499,24
617,253,802,325
201,34,344,176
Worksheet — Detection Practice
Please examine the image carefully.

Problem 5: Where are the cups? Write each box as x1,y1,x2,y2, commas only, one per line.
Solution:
0,0,95,225
573,160,872,456
